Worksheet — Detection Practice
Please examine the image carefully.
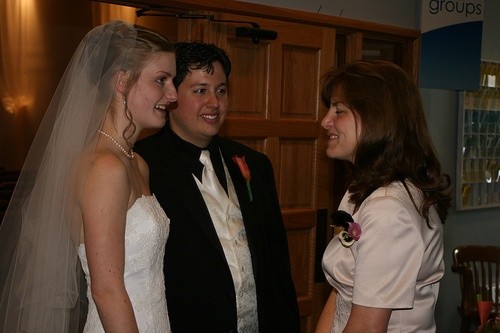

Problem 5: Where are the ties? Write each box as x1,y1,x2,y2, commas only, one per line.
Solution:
195,149,230,219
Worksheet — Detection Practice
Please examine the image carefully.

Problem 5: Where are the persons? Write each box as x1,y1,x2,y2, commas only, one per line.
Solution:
129,42,298,333
3,19,173,333
314,59,455,332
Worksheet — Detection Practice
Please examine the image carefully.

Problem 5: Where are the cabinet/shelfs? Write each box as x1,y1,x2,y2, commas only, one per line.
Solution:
455,61,500,210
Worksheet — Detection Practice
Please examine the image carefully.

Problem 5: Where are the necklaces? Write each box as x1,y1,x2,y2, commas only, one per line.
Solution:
97,127,135,161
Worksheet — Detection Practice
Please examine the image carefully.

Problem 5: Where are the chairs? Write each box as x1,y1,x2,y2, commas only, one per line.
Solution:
451,244,500,332
